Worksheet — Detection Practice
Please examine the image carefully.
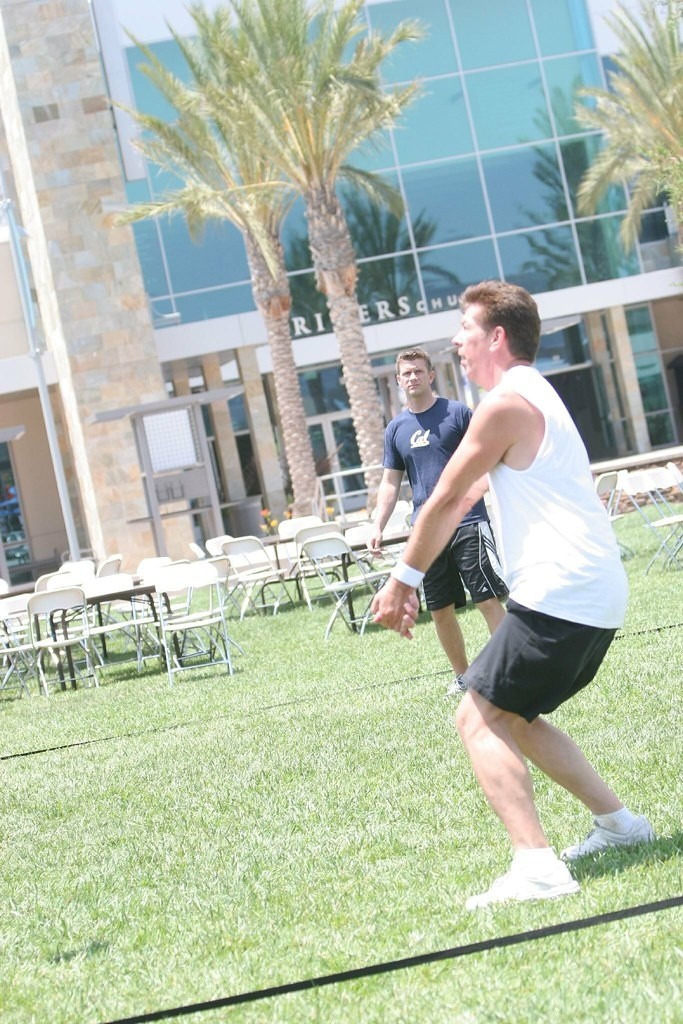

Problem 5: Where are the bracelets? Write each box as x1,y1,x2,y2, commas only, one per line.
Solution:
389,562,425,587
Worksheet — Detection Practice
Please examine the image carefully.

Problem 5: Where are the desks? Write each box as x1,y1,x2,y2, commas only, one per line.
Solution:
340,531,424,631
0,581,39,607
32,584,165,690
259,535,294,575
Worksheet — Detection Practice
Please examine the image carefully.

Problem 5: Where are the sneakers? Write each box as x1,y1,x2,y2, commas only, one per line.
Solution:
559,815,657,861
465,862,580,911
445,673,468,697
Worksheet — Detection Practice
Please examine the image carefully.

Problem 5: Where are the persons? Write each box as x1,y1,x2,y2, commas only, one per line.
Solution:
371,280,653,908
366,348,508,699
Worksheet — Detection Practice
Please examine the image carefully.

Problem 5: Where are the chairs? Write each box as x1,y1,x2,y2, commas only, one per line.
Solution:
595,462,683,577
0,499,425,698
